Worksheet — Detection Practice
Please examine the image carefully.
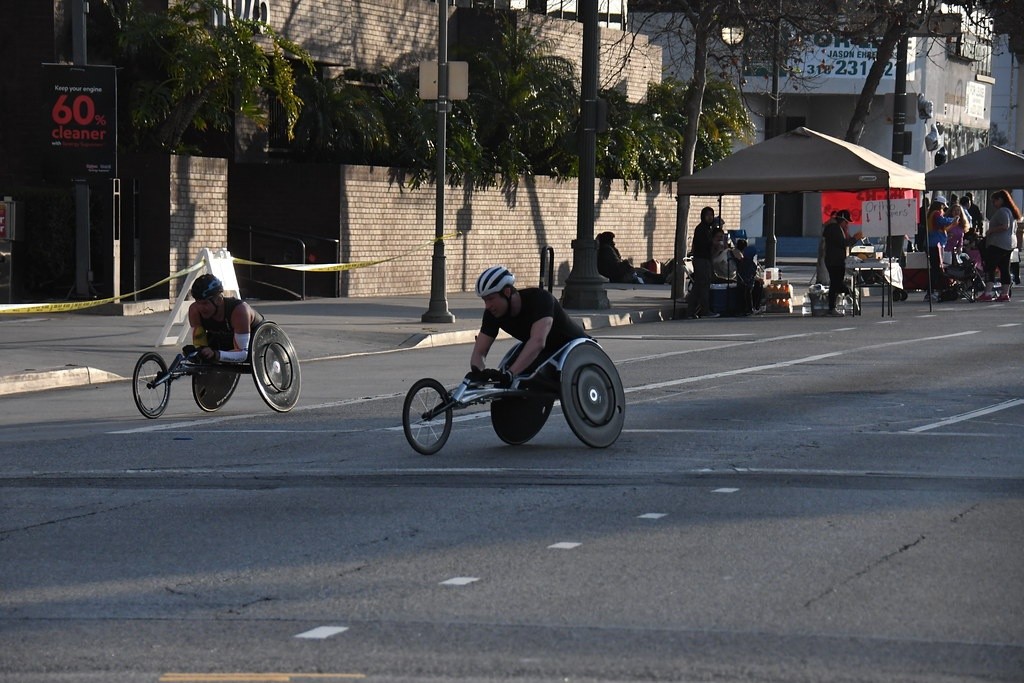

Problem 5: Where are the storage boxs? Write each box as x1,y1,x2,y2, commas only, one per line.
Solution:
904,252,933,268
709,281,739,315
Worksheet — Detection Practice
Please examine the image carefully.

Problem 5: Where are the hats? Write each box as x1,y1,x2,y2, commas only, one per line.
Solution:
934,196,949,208
836,210,853,222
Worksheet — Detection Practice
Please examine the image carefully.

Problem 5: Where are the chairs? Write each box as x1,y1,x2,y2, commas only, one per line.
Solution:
924,259,980,304
682,255,740,316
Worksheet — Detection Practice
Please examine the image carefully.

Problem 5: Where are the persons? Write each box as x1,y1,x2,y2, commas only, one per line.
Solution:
710,228,763,317
916,190,1022,303
463,267,600,396
692,207,728,316
823,208,863,316
597,231,663,286
178,274,272,363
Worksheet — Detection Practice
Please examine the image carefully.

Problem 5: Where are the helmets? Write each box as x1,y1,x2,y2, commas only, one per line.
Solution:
711,217,725,229
191,274,224,300
475,267,516,296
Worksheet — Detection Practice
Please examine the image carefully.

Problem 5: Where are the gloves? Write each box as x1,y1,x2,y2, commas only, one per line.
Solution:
480,368,513,388
463,366,483,390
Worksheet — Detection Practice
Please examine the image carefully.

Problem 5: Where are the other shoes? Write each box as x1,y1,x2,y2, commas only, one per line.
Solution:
924,293,938,301
700,311,720,317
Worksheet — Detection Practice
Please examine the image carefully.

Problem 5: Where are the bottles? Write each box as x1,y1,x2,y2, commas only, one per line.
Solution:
843,295,853,315
802,292,812,316
766,284,794,305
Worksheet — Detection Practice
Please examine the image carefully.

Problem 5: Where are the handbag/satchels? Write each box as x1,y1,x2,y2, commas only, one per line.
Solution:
1009,248,1019,262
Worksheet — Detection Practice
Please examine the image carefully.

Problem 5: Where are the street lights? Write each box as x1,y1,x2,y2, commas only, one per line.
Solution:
721,4,778,267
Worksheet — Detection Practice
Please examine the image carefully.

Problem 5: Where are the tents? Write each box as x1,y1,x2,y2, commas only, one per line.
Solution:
675,122,935,320
923,143,1024,302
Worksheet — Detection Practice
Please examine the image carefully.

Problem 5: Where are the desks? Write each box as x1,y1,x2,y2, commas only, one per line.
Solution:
902,268,929,290
844,258,903,317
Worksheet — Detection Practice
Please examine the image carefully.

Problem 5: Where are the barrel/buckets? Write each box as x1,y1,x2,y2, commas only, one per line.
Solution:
763,268,779,284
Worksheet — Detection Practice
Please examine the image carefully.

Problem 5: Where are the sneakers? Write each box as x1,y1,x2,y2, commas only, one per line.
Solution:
975,293,993,302
995,293,1011,302
827,308,844,317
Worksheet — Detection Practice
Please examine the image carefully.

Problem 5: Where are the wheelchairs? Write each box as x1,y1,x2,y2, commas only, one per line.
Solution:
402,339,629,456
133,320,302,419
